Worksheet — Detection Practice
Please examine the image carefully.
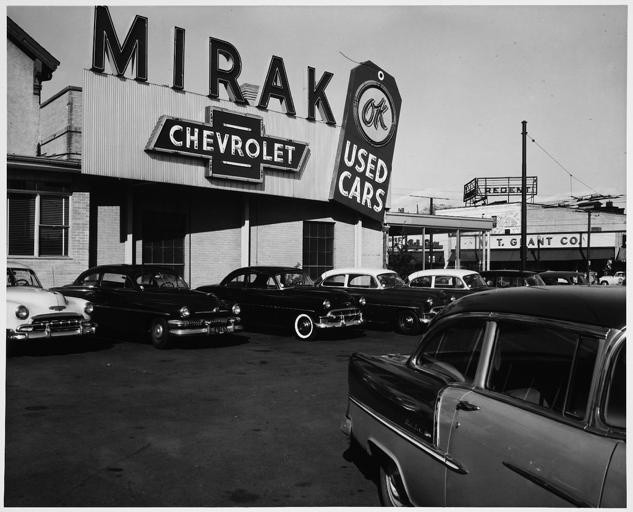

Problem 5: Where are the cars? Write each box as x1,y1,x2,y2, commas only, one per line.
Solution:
526,271,588,285
7,267,96,345
314,267,452,336
404,268,498,291
196,266,364,341
48,264,243,350
478,269,546,289
338,282,626,508
598,271,625,286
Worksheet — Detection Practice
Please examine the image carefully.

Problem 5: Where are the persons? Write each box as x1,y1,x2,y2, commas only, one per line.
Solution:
589,260,592,271
403,256,421,284
571,268,579,284
604,259,613,275
